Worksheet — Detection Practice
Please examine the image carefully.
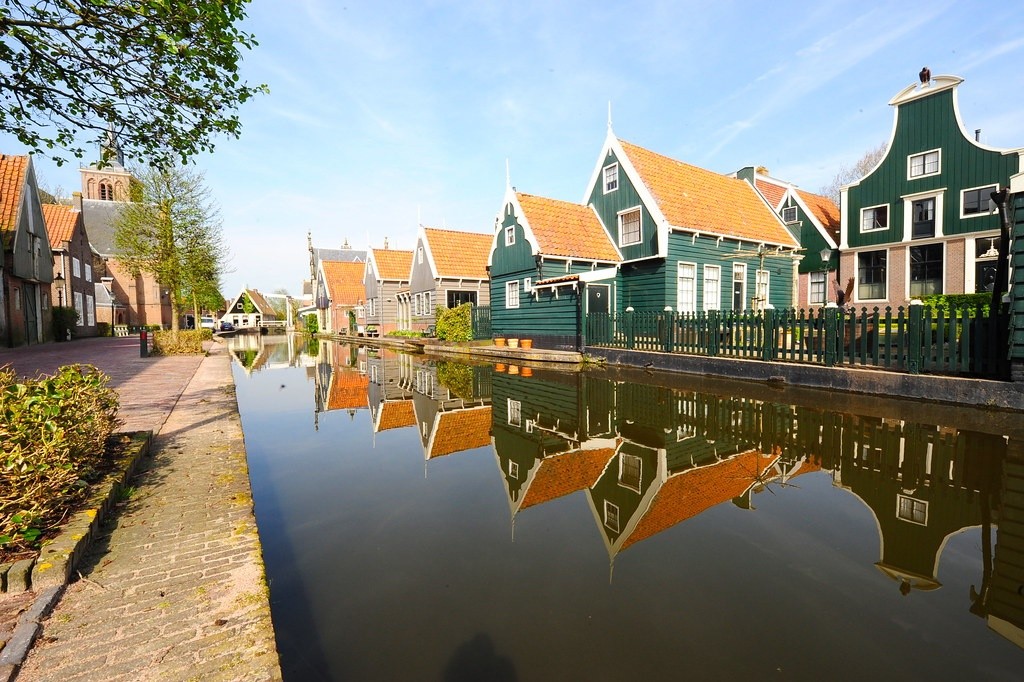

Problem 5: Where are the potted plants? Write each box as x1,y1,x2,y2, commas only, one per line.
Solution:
52,307,80,341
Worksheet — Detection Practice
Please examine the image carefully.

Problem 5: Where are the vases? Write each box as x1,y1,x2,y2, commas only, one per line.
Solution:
507,339,519,347
495,338,505,346
495,363,533,378
521,339,532,348
